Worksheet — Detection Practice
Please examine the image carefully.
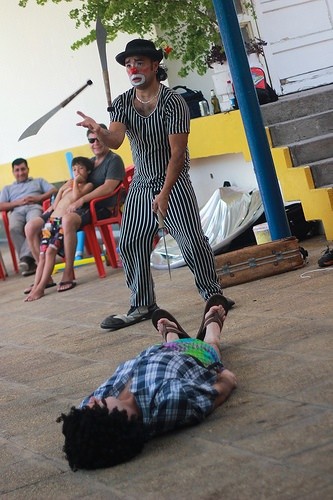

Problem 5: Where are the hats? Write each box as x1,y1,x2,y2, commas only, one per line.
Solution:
115,39,163,66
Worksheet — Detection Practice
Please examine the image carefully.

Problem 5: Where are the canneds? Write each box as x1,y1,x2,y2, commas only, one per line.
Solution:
199,101,210,117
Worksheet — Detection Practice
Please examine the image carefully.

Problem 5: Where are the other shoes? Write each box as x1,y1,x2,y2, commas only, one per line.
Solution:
222,296,235,307
22,262,37,276
19,256,32,270
99,303,160,329
318,245,333,267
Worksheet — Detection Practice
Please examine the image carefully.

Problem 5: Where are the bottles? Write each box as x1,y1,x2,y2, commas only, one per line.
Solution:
210,89,221,114
226,81,239,110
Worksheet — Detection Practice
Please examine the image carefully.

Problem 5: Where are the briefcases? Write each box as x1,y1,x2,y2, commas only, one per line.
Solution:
215,236,308,288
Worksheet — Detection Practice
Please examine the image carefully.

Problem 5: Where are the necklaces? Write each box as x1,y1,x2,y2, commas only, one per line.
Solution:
136,92,158,104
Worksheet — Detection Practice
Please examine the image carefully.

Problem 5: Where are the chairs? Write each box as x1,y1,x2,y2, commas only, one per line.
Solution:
76,164,135,279
2,197,51,273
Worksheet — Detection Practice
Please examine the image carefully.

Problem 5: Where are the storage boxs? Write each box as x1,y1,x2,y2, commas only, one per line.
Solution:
214,236,304,288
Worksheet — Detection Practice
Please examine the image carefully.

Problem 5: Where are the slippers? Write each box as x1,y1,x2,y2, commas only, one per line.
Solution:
196,295,229,340
57,281,76,291
24,282,56,294
153,309,191,342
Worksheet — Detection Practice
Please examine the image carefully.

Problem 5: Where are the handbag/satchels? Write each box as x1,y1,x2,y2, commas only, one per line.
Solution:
232,68,278,109
172,86,209,119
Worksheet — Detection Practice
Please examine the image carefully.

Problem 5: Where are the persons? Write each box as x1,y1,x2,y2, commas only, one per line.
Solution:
0,158,58,275
23,123,126,302
56,294,236,472
77,40,235,331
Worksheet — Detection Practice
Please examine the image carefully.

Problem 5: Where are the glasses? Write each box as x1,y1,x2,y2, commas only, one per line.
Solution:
88,138,99,143
